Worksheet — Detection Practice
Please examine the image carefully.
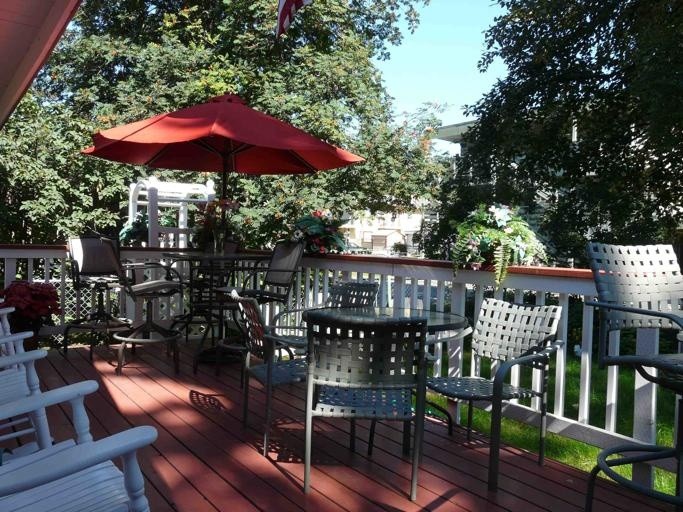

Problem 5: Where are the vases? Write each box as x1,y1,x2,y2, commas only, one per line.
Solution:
10,325,39,351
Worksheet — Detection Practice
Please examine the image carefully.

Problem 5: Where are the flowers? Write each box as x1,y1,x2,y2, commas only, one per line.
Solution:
0,279,62,331
444,199,545,286
283,211,350,253
184,200,265,253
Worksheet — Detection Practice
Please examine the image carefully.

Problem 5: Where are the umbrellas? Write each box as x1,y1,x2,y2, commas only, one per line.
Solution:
79,93,366,340
274,0,313,39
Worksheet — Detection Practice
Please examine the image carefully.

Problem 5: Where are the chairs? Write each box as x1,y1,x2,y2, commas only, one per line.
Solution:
303,312,431,503
230,288,356,459
405,296,563,492
0,296,160,511
584,241,682,511
273,282,380,363
66,230,307,390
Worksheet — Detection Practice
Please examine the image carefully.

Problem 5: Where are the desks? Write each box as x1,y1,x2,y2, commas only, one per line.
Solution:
304,306,469,501
